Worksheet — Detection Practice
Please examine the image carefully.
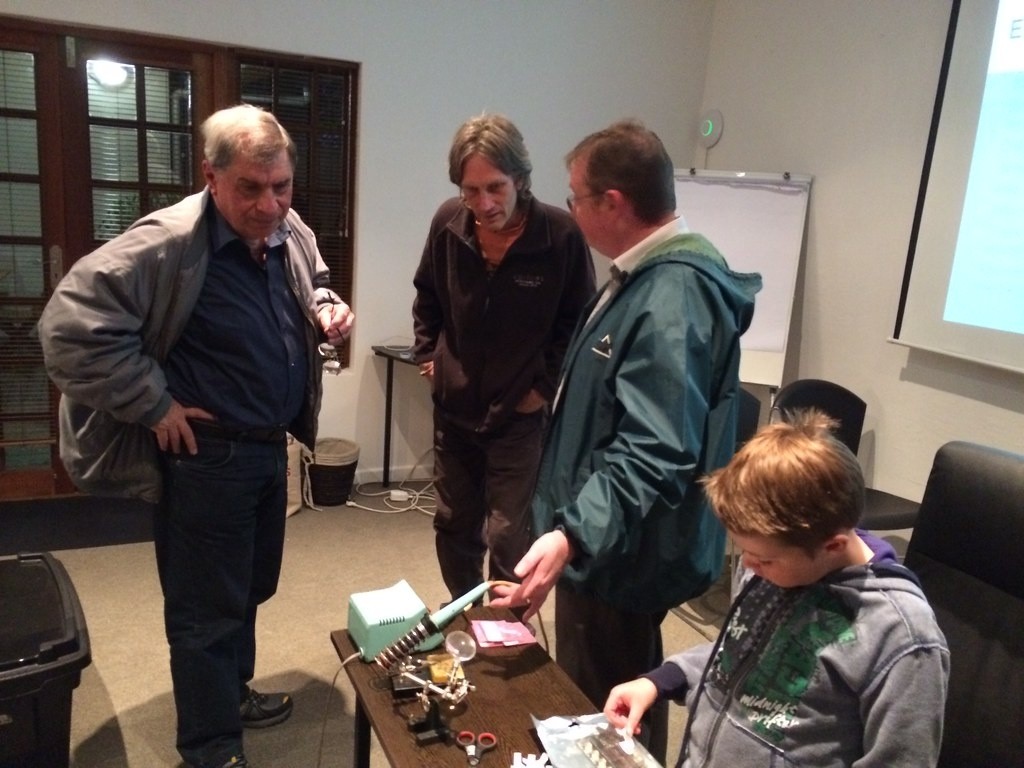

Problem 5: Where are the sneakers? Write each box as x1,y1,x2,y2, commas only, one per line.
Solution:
236,690,292,728
218,753,250,768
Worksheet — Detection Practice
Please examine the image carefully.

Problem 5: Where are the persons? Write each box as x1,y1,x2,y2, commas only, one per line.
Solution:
35,101,355,768
490,119,762,768
411,112,599,636
604,405,953,768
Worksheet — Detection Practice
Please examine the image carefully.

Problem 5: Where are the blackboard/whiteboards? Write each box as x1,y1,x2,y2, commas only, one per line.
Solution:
672,167,813,389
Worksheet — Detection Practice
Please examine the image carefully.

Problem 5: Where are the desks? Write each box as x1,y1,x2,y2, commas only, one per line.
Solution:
332,602,667,768
371,343,417,486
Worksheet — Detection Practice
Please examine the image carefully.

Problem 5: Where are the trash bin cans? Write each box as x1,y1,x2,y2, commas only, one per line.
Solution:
0,551,92,768
302,435,361,507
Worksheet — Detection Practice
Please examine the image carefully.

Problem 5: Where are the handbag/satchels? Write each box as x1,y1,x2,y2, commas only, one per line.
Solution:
286,435,303,518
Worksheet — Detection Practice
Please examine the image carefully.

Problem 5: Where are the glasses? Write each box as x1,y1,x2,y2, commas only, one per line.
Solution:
567,191,608,210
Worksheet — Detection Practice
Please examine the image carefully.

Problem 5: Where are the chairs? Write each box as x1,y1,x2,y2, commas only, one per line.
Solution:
900,441,1024,768
767,376,924,536
